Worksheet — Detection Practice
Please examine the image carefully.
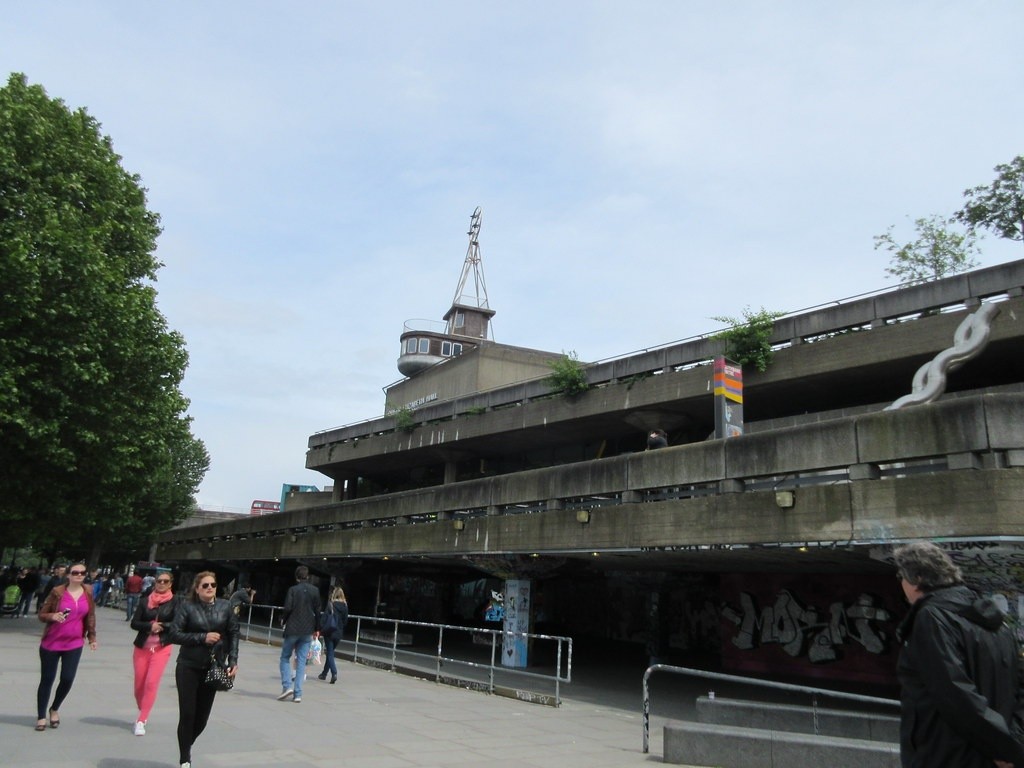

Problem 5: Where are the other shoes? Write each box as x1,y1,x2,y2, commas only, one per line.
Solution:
330,674,337,684
318,671,326,680
36,724,46,730
293,698,301,702
277,688,294,700
181,762,191,768
49,707,60,728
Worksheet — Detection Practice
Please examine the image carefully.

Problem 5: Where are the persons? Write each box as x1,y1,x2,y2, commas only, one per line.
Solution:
35,561,98,731
319,586,350,686
275,565,323,703
891,539,1024,768
229,581,257,622
169,571,240,768
131,571,182,735
0,564,157,621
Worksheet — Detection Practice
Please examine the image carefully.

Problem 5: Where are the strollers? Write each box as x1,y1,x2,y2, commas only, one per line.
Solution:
0,584,24,618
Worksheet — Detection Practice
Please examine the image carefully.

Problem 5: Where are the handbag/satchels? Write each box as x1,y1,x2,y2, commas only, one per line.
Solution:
306,634,321,666
323,614,338,630
205,664,233,691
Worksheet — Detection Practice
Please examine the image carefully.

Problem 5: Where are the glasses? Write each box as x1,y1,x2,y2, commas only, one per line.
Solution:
156,579,170,584
199,583,217,588
70,570,87,576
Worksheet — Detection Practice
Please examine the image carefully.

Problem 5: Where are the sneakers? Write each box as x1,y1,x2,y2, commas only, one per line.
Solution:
135,720,146,736
